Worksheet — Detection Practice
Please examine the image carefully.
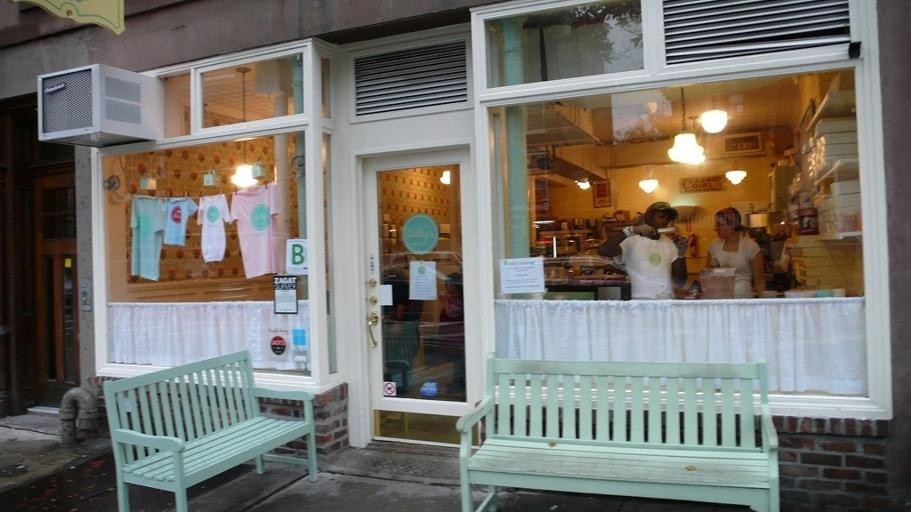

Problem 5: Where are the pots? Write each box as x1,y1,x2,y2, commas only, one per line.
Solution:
572,217,585,229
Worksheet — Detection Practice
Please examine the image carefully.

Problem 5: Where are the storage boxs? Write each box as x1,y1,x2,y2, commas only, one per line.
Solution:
101,349,320,512
456,357,782,512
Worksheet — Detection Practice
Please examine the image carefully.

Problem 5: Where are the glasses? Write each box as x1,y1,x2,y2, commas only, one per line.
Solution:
666,88,707,168
637,130,660,195
698,95,728,134
573,144,591,191
439,170,451,185
724,146,748,186
232,66,259,189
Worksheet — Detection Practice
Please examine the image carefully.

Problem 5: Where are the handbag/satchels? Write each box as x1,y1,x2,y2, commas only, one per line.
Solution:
621,223,634,238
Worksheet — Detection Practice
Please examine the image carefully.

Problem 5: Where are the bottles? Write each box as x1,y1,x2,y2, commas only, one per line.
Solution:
645,202,678,219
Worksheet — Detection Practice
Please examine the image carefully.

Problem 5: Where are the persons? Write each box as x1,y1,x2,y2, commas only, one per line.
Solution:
382,268,424,387
438,273,464,393
752,236,791,294
705,207,768,299
597,202,690,301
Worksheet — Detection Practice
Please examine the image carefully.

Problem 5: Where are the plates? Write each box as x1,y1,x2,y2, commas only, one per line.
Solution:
767,166,794,212
524,100,600,150
802,88,862,242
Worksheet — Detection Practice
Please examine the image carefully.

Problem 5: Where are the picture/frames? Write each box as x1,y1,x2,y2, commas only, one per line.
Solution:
545,278,631,300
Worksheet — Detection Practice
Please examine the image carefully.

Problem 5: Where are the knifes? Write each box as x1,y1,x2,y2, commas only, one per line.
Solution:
36,64,165,147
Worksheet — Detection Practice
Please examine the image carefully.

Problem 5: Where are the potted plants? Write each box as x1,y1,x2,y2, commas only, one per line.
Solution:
722,131,764,153
592,178,612,208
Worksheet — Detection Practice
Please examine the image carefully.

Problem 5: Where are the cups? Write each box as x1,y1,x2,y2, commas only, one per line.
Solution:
713,222,728,226
